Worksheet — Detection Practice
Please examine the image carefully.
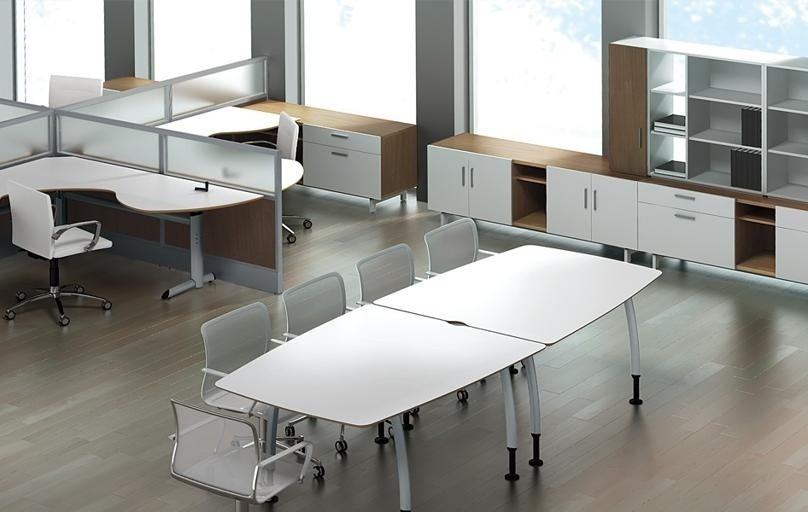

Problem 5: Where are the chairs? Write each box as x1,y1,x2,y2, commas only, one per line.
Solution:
424,218,519,385
356,243,469,416
281,272,391,453
5,181,115,329
200,303,325,480
169,397,313,511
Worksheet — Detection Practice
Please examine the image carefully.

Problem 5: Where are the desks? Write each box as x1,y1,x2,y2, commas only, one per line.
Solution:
372,244,662,467
214,302,547,511
1,74,312,296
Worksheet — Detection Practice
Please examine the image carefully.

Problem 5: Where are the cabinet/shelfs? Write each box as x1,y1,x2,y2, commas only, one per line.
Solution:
608,33,808,203
104,77,247,105
637,175,808,284
427,131,649,263
236,99,418,215
